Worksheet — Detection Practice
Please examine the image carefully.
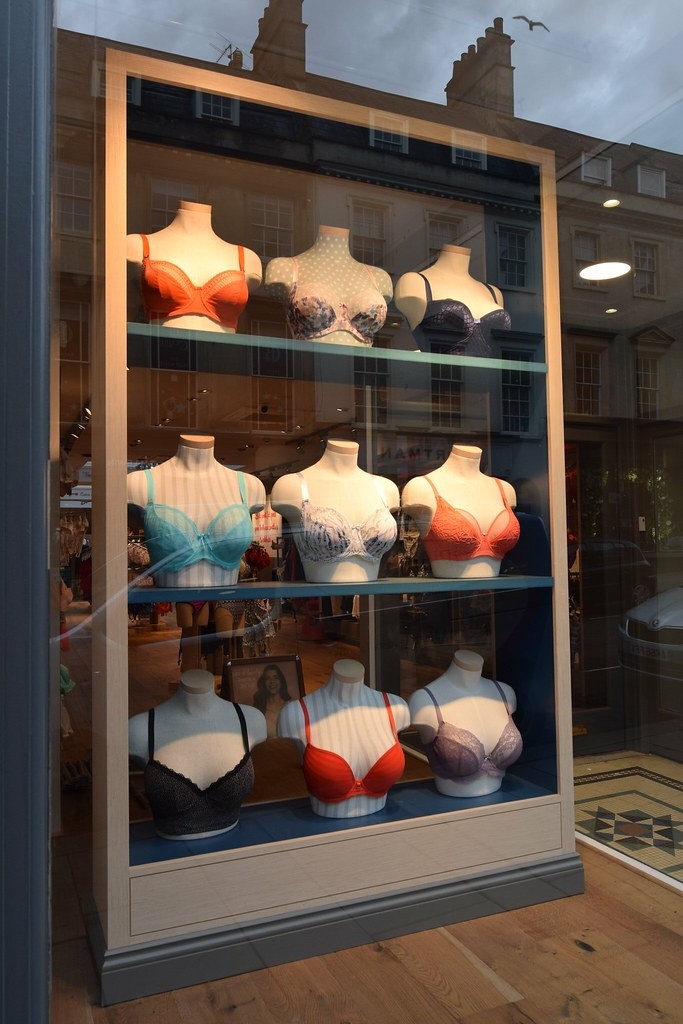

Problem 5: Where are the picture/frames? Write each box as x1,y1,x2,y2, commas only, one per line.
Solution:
225,655,306,736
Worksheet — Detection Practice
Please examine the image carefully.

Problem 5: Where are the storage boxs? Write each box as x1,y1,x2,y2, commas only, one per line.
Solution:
339,620,359,640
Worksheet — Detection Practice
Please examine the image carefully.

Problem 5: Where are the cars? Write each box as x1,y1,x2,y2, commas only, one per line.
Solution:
569,539,660,618
613,583,683,697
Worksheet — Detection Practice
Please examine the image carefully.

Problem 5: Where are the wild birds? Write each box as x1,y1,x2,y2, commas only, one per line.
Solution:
512,15,550,33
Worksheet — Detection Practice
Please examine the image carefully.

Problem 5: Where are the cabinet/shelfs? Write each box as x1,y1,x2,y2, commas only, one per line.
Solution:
86,46,585,1005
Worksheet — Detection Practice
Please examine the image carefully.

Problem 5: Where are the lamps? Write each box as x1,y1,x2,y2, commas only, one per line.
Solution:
349,423,358,441
70,418,89,439
296,440,305,455
319,436,326,450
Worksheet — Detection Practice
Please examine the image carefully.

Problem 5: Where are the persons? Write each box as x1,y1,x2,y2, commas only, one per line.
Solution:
252,663,294,741
125,195,523,843
